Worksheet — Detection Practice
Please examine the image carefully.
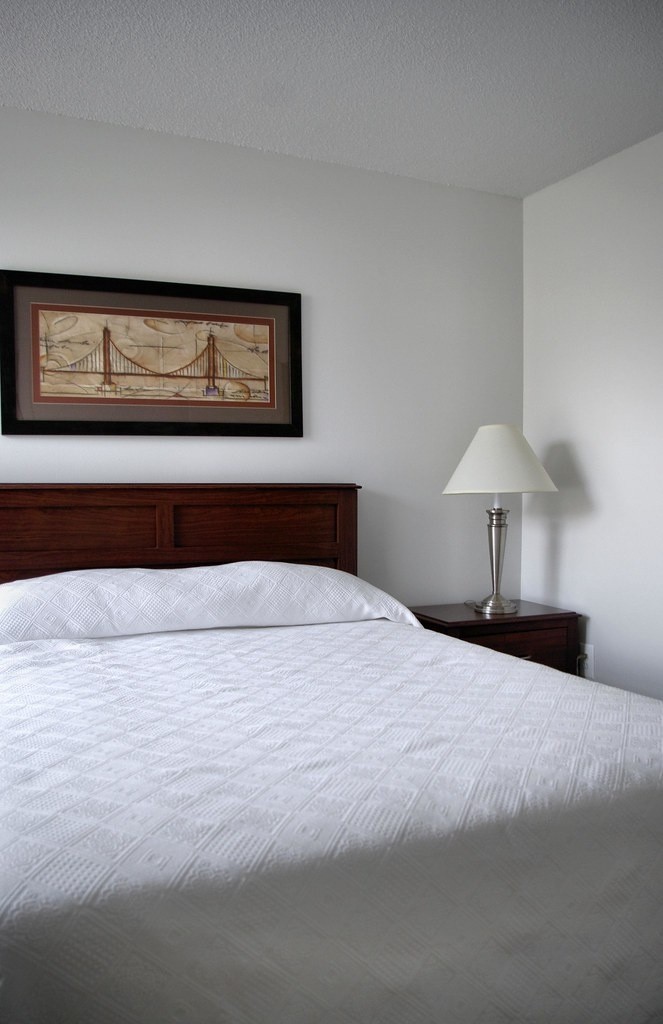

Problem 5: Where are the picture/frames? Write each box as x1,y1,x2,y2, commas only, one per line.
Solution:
0,269,304,438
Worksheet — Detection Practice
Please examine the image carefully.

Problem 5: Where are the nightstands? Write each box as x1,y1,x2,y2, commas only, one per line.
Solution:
408,599,575,678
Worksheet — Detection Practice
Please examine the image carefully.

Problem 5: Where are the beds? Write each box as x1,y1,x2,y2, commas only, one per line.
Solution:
0,483,663,1024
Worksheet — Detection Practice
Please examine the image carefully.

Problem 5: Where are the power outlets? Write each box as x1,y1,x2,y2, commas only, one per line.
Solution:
581,643,594,679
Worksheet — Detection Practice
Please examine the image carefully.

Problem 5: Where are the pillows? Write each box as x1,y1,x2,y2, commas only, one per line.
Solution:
0,560,424,644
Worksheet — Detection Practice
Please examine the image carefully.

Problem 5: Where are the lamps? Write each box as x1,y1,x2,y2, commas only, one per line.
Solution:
442,425,558,614
